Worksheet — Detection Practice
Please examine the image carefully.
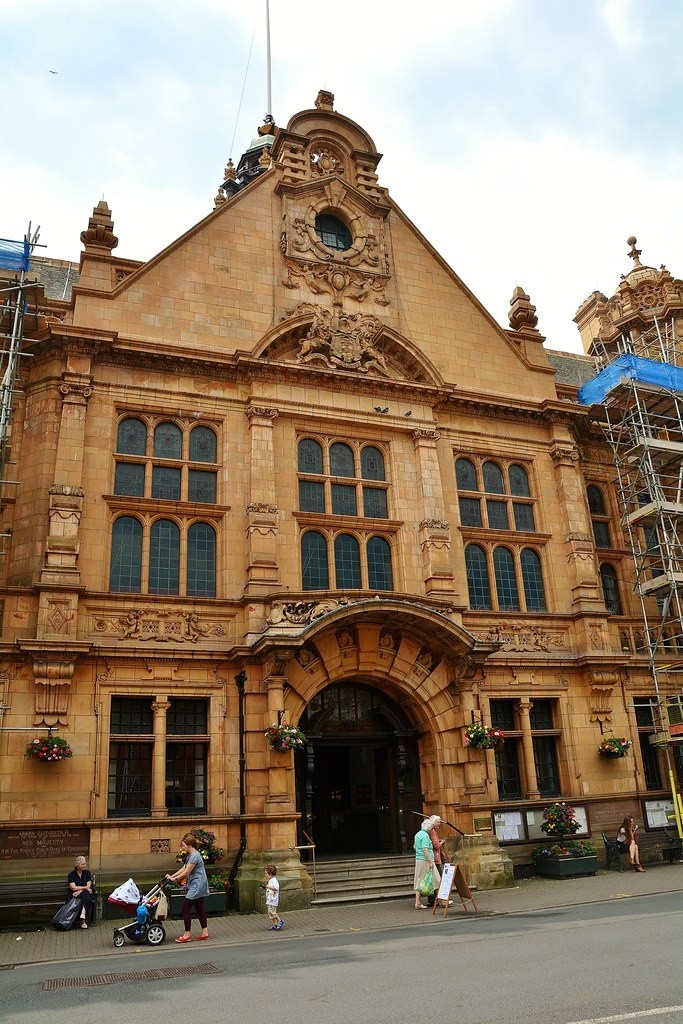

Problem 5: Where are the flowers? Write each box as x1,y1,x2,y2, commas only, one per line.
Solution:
530,801,594,857
167,828,236,893
24,735,73,759
598,737,631,756
265,722,308,749
463,722,503,749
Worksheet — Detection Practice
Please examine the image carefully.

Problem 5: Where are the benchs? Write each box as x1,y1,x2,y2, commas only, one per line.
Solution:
601,828,681,873
0,871,99,929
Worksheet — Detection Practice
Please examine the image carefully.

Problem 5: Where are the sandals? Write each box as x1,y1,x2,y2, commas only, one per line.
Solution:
414,904,427,909
269,924,277,930
277,920,284,928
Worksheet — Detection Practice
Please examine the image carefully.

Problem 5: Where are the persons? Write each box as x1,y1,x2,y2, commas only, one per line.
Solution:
165,835,210,942
258,864,284,931
413,819,453,910
616,815,646,872
428,815,454,906
65,856,93,929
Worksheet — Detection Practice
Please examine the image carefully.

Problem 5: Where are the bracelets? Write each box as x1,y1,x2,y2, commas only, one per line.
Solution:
175,877,178,880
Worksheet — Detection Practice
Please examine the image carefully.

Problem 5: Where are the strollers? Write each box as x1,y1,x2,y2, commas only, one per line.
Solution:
108,877,172,948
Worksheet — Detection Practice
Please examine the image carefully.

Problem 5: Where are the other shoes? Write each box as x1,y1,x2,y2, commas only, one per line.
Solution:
174,935,192,942
80,913,86,919
195,935,209,941
81,922,88,928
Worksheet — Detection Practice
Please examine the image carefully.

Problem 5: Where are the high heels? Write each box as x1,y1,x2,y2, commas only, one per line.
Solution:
629,858,636,866
635,864,645,872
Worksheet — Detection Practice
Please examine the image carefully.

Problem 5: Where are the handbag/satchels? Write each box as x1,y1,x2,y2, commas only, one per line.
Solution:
415,868,434,896
155,891,168,921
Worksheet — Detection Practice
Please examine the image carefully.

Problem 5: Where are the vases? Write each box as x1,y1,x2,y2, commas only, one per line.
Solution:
172,889,226,920
470,735,494,749
602,750,624,759
39,752,60,763
546,853,599,879
274,738,294,752
